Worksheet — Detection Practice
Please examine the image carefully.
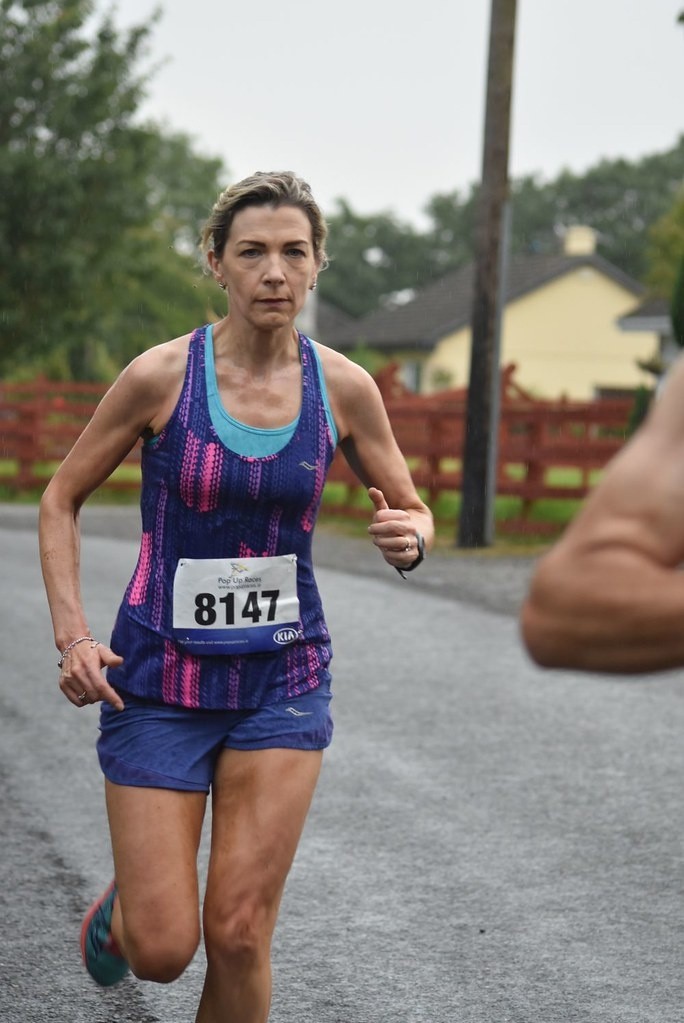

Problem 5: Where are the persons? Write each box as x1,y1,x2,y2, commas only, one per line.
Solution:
521,347,684,674
39,172,434,1023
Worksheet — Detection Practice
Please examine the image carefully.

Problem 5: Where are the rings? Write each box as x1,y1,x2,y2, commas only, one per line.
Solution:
403,536,410,552
78,691,85,700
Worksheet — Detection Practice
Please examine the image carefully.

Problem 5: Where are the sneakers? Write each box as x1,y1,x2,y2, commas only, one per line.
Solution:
80,880,130,989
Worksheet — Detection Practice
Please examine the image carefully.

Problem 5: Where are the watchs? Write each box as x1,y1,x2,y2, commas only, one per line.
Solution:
395,529,426,580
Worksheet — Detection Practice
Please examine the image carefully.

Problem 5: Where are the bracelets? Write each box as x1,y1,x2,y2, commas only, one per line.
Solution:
58,637,99,669
